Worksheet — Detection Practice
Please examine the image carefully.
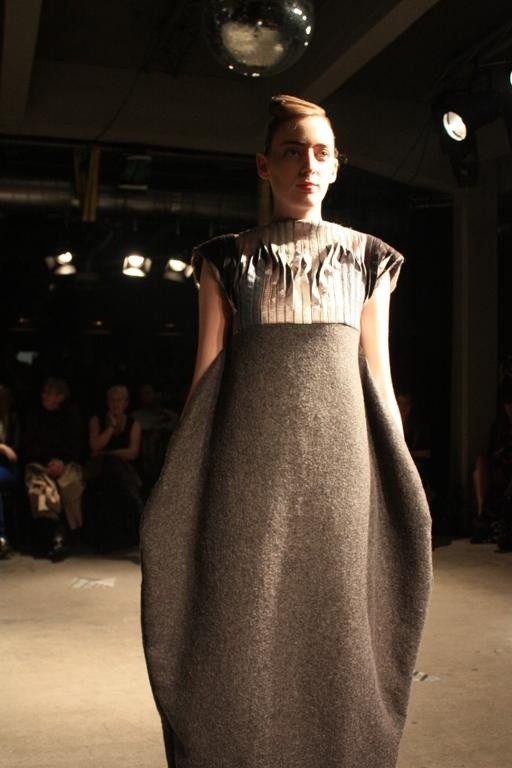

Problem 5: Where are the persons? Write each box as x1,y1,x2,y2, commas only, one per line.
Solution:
1,371,179,561
395,387,436,492
140,94,434,768
471,400,510,543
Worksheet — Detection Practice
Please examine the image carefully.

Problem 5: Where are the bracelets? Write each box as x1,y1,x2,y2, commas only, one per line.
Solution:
109,424,116,429
